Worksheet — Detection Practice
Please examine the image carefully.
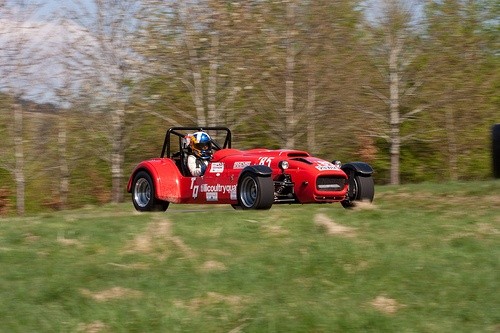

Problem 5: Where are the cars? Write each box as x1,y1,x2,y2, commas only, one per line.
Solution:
127,126,375,212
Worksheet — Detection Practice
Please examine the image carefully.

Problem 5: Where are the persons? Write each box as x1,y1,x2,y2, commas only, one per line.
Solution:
187,132,213,176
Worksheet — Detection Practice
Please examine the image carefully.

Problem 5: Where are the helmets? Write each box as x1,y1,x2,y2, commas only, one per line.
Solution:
190,132,213,159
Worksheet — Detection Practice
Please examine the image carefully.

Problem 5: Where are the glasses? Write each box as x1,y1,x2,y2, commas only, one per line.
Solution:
335,162,342,166
